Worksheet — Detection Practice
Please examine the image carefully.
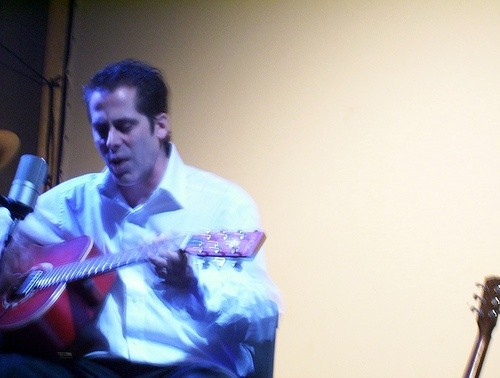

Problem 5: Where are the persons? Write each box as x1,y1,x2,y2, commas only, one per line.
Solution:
0,58,279,378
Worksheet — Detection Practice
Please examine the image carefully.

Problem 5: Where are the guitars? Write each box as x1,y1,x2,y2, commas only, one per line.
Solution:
461,277,500,378
0,229,267,350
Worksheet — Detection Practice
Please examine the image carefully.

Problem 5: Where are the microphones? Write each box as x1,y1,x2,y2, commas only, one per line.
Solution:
3,154,47,248
